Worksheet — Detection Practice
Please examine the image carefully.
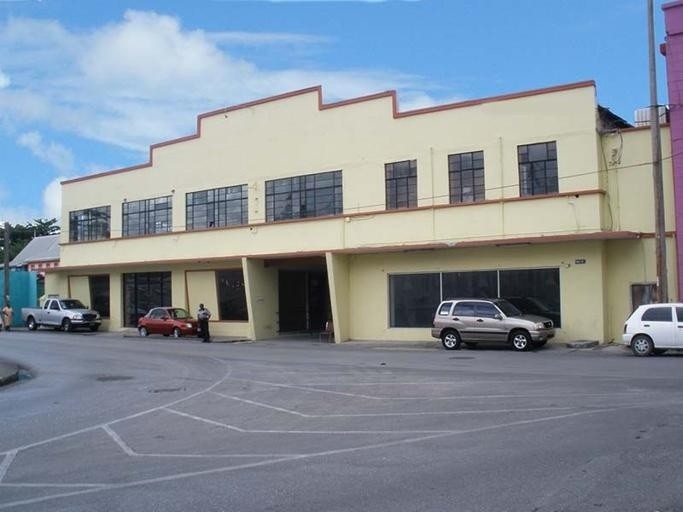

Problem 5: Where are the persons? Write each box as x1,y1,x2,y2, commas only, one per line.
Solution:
197,303,211,343
3,303,14,331
0,309,3,331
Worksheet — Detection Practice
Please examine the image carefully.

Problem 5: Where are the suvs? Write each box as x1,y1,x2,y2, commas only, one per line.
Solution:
621,301,683,358
502,295,559,325
431,298,557,353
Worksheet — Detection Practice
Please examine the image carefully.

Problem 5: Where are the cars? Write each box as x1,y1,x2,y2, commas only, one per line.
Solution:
137,306,200,340
129,304,146,324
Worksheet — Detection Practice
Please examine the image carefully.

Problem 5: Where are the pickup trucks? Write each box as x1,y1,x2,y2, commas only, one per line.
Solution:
21,294,104,335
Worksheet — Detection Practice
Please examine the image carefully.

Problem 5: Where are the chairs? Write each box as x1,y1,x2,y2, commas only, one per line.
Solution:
319,320,334,343
